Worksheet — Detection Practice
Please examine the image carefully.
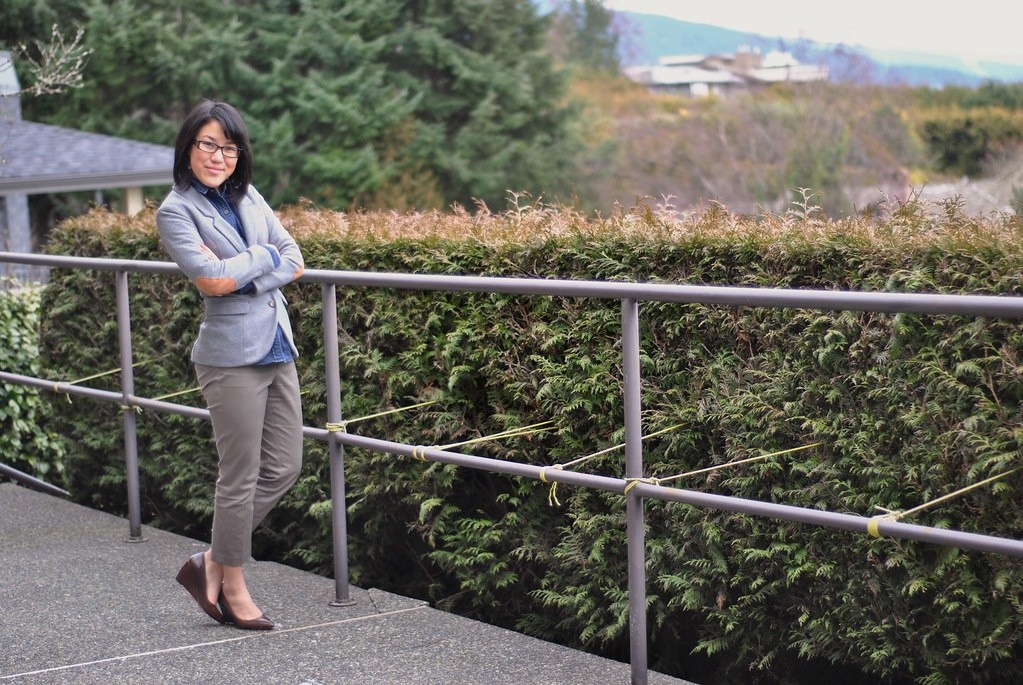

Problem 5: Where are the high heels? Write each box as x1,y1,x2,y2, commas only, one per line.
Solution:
215,583,274,629
176,553,226,625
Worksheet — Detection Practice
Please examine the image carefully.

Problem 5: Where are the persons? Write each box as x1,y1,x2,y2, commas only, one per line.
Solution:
156,101,305,630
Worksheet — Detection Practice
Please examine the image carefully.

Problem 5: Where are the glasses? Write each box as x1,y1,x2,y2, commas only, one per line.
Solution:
191,138,243,158
